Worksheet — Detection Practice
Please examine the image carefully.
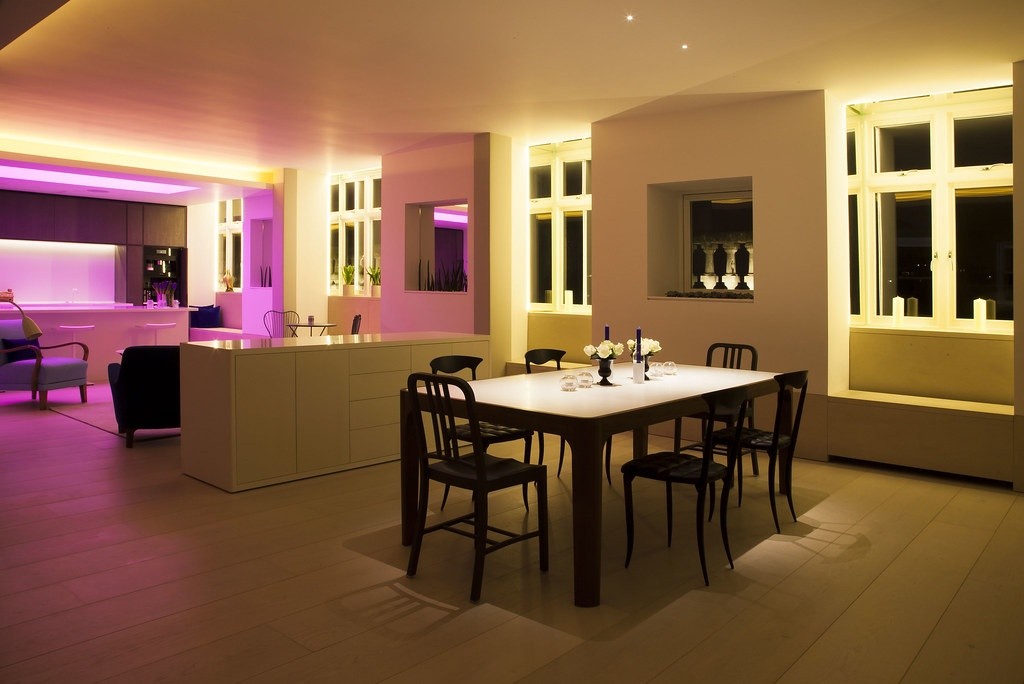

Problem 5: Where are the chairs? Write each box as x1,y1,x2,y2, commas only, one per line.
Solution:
263,310,362,339
0,319,90,410
405,343,807,603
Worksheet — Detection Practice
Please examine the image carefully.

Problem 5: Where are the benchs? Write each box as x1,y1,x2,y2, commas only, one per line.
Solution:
189,328,242,342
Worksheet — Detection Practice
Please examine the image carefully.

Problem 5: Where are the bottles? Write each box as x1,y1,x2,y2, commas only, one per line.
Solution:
144,281,152,302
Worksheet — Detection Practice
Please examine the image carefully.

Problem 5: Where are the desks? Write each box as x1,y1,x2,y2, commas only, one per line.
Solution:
285,324,337,338
401,363,786,609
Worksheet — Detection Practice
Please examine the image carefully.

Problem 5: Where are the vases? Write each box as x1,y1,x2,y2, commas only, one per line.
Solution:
157,293,174,307
597,360,613,386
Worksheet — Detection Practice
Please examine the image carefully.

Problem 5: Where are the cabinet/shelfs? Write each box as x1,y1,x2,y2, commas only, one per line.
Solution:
176,330,492,493
0,189,187,249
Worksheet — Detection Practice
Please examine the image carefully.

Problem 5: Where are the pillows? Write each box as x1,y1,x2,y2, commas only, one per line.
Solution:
189,304,221,328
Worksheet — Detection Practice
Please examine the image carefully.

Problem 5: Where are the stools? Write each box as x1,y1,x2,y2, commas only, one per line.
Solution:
60,322,178,386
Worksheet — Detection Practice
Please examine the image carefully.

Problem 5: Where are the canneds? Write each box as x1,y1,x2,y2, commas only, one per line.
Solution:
308,316,314,325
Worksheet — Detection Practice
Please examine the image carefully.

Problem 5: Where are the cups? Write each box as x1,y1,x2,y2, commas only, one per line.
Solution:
577,372,593,388
156,294,174,307
561,375,578,391
147,300,154,308
663,361,677,375
173,300,179,308
649,362,663,377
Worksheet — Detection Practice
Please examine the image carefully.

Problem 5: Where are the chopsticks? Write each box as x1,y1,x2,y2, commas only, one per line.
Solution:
153,281,176,294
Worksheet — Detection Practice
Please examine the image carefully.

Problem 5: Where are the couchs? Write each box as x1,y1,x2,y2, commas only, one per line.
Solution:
108,345,181,449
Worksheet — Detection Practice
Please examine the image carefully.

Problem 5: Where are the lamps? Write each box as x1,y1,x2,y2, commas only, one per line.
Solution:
220,270,236,293
0,289,43,341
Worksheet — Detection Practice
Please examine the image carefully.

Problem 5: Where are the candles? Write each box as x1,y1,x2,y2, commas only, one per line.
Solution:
545,290,552,302
986,298,996,319
636,326,641,363
892,294,904,328
973,297,986,332
605,324,609,341
565,290,573,313
907,296,918,316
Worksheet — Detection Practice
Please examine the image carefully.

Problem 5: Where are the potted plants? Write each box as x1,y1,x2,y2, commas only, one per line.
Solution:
366,267,382,298
342,265,355,297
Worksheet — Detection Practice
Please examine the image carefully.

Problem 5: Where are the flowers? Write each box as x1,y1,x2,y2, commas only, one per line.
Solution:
583,338,662,359
152,281,177,293
